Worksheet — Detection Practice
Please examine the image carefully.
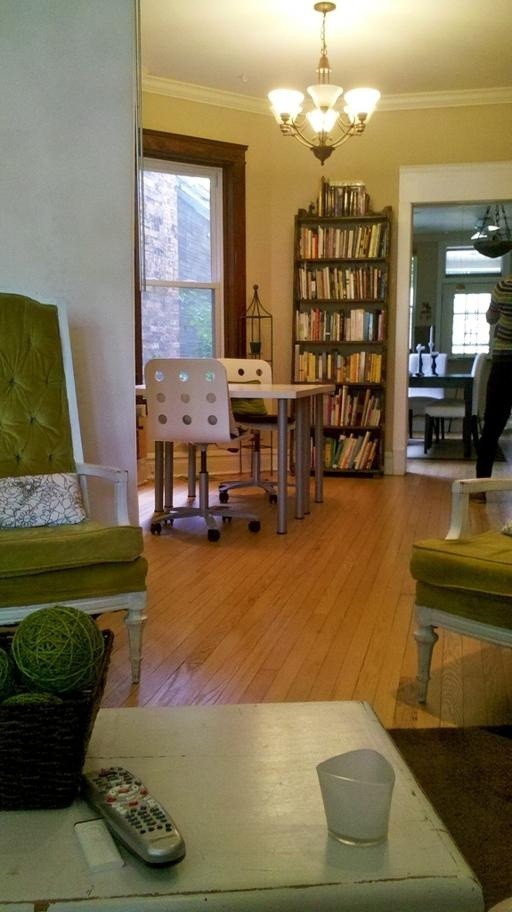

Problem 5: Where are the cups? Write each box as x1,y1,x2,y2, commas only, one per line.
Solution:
315,747,396,846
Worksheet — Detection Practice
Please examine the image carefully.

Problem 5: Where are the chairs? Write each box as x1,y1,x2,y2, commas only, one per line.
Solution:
1,291,147,690
409,353,447,441
146,358,262,538
215,357,297,503
408,476,512,709
421,353,487,451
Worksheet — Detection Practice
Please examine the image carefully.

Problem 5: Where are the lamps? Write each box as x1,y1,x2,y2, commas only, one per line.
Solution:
267,2,385,166
474,203,512,258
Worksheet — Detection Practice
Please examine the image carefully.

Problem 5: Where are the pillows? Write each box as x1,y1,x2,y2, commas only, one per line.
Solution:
2,472,86,528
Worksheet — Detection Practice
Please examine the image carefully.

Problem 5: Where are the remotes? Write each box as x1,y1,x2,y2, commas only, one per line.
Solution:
84,764,188,871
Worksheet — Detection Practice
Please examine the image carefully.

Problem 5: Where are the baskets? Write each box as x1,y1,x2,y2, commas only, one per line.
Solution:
0,625,114,810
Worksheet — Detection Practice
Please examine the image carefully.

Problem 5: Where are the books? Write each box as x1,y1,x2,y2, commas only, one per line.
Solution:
289,175,390,471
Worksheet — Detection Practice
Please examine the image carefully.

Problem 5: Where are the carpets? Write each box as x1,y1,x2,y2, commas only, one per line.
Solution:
389,723,511,910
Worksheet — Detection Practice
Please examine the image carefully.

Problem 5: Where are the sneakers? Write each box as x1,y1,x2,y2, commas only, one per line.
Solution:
470,491,487,505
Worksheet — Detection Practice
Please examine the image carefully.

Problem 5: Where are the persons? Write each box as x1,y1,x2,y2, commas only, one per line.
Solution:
467,277,512,505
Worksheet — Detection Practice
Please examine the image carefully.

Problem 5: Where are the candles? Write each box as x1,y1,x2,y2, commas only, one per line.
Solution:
429,326,434,343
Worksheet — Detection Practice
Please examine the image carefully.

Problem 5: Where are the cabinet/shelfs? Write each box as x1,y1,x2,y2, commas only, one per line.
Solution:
292,208,395,478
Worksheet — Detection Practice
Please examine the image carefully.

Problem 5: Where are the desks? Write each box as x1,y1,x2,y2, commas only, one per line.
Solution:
0,695,486,907
409,376,474,460
138,384,334,535
